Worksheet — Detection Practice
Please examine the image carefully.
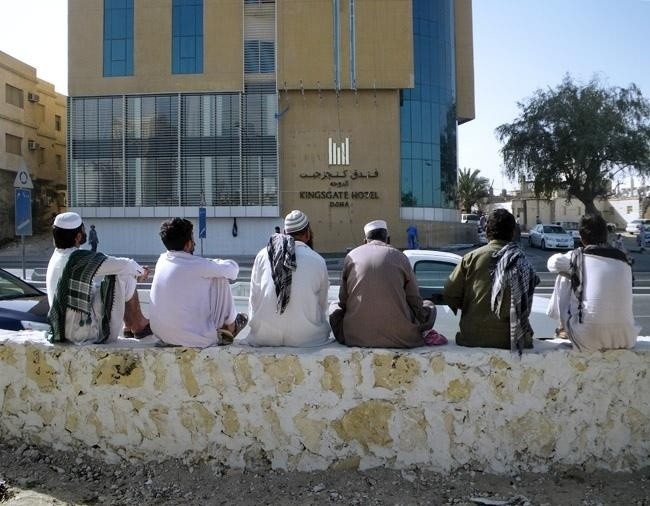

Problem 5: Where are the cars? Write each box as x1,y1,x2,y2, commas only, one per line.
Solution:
461,214,480,225
625,219,650,235
0,269,50,332
528,223,574,251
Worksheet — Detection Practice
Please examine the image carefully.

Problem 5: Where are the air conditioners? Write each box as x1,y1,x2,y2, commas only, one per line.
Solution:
28,92,41,102
29,141,41,150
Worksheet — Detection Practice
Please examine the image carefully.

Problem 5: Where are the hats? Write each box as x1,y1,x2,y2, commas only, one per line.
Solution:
364,220,387,236
53,212,83,230
284,210,309,234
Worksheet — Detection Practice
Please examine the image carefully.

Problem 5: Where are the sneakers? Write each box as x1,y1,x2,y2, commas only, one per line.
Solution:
555,328,568,339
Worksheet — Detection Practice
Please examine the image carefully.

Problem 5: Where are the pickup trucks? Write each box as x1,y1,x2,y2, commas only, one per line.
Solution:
226,248,563,346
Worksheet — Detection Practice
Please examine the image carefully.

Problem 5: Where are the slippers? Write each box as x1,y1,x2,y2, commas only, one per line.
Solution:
123,331,134,338
134,319,153,339
232,312,249,337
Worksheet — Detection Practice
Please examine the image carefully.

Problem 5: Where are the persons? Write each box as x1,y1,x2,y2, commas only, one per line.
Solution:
148,215,248,349
479,213,487,229
406,219,420,250
443,208,540,349
516,214,521,237
45,209,154,343
247,207,328,348
612,234,625,249
330,218,437,348
638,221,645,247
536,216,542,224
273,226,281,234
87,224,100,252
546,213,642,353
